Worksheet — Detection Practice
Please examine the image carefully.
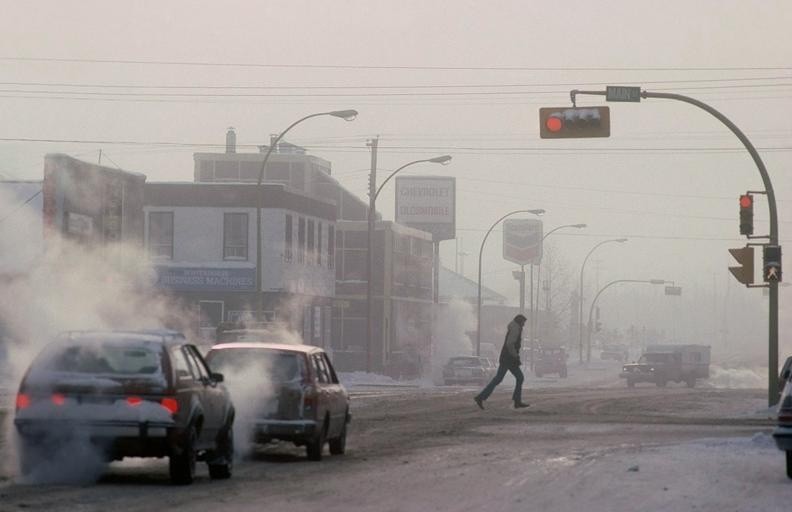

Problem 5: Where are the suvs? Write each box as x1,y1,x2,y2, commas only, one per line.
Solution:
14,325,236,483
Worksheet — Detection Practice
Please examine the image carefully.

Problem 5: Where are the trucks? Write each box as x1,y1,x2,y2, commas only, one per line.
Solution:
619,343,711,389
535,347,568,378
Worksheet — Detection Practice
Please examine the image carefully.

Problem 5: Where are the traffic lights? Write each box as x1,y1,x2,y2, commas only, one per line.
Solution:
728,247,753,284
762,244,783,283
740,194,754,236
539,106,611,139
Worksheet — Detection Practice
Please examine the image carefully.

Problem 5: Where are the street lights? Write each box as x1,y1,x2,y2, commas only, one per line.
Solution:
476,208,546,357
254,109,359,326
365,154,452,372
528,222,588,370
578,237,629,364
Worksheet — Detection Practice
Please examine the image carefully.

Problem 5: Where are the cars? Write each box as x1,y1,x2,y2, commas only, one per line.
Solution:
442,355,497,387
202,343,352,462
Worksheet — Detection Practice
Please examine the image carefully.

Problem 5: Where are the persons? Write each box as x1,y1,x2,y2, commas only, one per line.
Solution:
473,313,532,410
